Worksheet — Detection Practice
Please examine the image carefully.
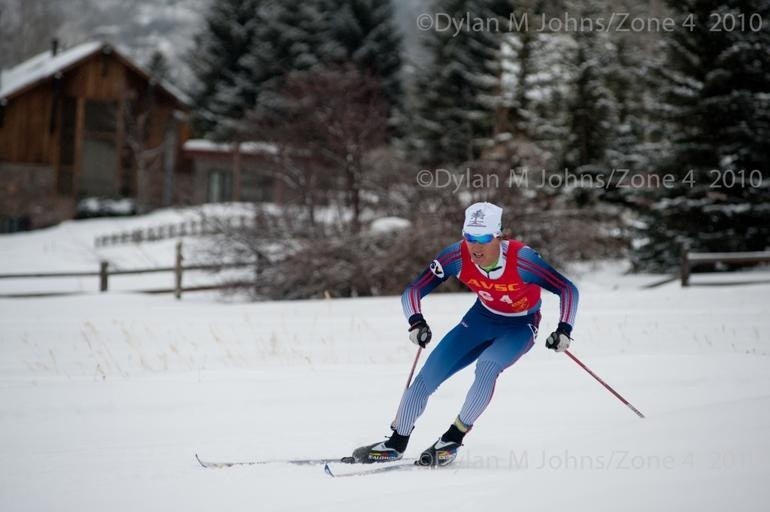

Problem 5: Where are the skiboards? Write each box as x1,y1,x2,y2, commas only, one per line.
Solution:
195,452,457,477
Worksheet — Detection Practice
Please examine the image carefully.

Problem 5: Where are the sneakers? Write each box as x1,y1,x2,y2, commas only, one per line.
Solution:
421,438,461,466
353,441,404,463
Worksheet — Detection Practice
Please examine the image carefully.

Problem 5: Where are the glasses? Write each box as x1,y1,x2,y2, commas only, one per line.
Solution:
464,232,494,243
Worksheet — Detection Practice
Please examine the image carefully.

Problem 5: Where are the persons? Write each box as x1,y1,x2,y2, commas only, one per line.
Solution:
354,201,579,469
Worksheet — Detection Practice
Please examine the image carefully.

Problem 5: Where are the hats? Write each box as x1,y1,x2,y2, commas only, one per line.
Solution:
462,203,503,239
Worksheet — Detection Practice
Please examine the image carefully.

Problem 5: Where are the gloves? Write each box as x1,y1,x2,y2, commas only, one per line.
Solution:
545,322,574,353
408,313,432,348
